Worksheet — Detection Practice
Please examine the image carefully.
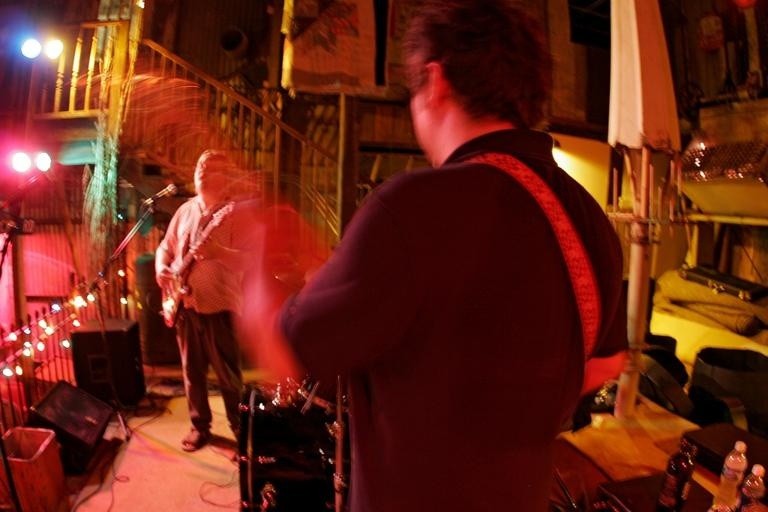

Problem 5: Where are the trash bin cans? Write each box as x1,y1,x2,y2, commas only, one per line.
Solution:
1,426,66,512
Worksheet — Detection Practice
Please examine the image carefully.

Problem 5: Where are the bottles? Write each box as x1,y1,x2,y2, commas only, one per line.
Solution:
713,440,748,512
657,438,697,511
741,463,768,512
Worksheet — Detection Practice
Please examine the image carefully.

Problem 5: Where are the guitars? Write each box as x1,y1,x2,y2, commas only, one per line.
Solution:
162,200,235,327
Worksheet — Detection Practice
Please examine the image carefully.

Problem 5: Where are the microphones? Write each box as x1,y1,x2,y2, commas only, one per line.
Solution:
143,184,178,208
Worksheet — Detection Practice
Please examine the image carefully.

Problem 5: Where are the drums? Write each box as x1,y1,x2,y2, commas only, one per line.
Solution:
233,377,352,511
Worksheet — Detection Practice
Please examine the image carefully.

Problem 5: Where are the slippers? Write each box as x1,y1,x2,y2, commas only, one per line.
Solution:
182,430,210,452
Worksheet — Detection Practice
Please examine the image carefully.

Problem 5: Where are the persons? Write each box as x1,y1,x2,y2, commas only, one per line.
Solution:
273,2,623,512
154,150,265,462
